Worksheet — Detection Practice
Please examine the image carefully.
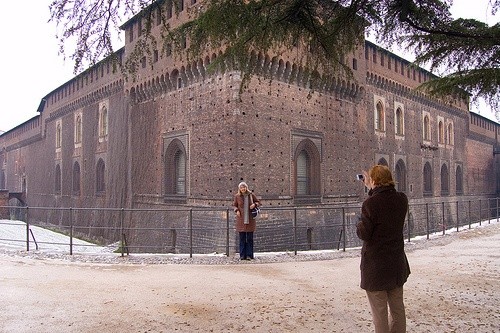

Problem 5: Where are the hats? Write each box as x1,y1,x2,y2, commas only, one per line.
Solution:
239,182,248,188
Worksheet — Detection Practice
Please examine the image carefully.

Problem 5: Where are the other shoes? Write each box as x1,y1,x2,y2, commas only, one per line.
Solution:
241,256,251,260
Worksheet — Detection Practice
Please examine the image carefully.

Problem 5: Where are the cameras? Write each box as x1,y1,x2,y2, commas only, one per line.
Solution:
357,174,364,181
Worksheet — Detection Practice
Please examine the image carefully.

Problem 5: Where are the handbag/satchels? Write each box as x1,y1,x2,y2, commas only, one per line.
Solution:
250,192,260,219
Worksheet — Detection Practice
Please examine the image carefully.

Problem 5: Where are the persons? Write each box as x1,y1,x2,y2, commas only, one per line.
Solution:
356,165,410,333
233,182,261,261
